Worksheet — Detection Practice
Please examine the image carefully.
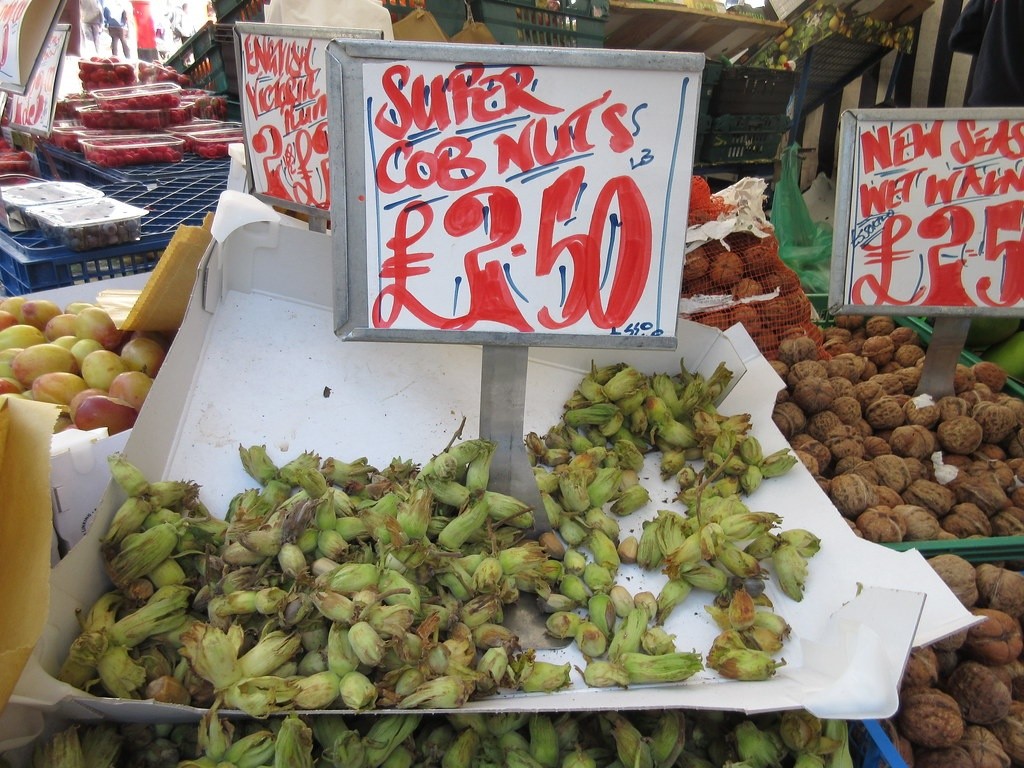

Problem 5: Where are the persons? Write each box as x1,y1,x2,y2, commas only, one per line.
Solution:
104,0,131,58
79,0,104,56
948,0,1023,107
177,4,199,45
132,0,162,63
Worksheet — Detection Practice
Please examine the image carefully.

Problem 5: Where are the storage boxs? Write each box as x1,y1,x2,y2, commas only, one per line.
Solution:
1,0,933,297
877,313,1024,562
849,719,908,768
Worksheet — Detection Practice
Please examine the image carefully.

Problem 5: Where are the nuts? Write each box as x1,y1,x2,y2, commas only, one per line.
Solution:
680,234,1023,767
